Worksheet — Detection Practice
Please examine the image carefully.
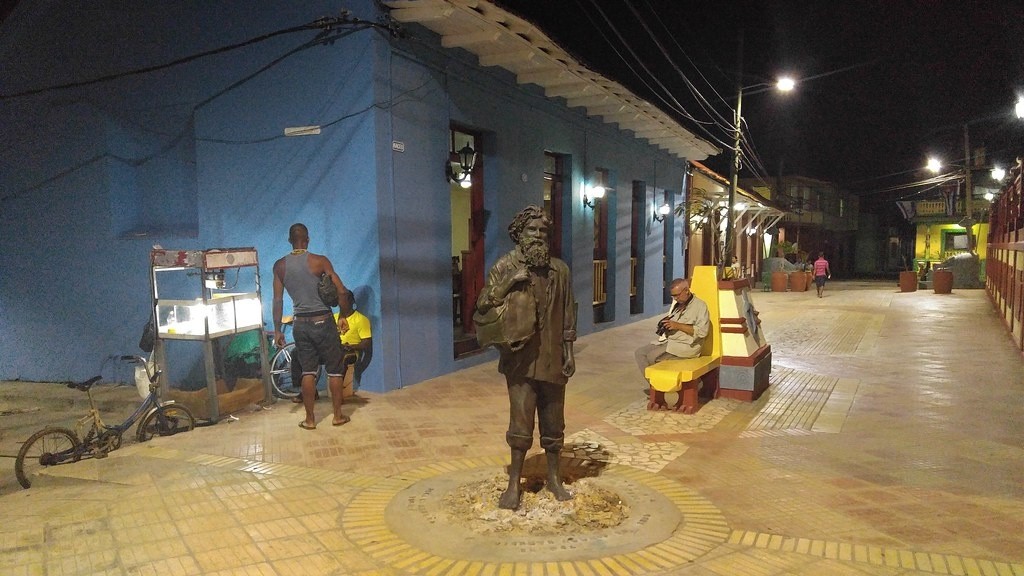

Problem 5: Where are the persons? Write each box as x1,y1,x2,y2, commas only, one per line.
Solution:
794,257,814,270
290,289,373,404
271,222,351,430
730,255,741,279
474,202,578,510
812,251,831,298
635,278,711,396
918,262,925,274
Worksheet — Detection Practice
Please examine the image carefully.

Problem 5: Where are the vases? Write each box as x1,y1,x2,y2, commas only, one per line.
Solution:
789,271,807,292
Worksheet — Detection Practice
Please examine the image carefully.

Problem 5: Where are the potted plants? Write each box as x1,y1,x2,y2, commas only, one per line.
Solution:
771,238,800,292
801,251,812,292
899,255,917,292
933,251,954,294
673,186,767,357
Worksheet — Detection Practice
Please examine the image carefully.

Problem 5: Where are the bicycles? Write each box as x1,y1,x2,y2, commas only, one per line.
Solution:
13,353,196,488
264,315,322,399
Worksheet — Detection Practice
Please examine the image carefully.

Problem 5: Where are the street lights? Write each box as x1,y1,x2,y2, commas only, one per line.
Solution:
928,160,973,253
723,78,795,280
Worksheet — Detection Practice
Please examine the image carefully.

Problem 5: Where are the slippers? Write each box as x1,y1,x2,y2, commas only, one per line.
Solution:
298,420,315,429
332,415,350,426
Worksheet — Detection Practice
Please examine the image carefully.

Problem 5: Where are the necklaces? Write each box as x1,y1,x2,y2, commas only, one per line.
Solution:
291,249,307,254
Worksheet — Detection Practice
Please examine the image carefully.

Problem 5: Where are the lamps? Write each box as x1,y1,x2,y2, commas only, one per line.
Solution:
583,184,605,210
653,204,670,223
445,141,477,184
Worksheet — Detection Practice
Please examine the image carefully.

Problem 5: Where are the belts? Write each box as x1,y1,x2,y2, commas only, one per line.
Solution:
294,312,333,322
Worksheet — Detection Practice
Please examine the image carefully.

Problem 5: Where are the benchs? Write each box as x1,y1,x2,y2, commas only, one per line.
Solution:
645,356,720,415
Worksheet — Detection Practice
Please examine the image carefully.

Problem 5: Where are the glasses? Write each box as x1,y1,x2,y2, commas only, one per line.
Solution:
670,287,687,298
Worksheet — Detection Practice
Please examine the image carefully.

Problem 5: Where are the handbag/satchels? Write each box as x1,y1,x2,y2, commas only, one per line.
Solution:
318,274,338,308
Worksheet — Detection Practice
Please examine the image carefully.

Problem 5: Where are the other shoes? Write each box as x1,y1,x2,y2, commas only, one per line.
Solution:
293,389,319,403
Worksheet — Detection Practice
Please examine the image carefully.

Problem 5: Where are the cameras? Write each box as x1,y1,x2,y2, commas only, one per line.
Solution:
656,319,670,336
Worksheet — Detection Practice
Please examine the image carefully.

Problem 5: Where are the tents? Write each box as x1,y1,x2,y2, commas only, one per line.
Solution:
689,159,788,238
